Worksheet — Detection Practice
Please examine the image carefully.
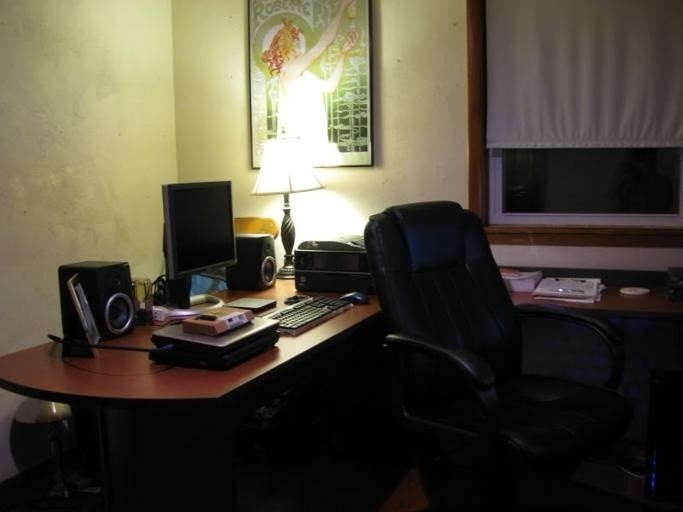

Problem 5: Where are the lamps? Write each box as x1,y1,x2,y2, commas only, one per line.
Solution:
250,126,325,280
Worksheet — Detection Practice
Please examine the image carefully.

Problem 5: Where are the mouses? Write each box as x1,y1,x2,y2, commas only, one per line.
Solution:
339,292,369,305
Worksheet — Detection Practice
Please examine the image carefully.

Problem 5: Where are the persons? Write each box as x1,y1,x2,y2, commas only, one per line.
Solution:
261,0,360,155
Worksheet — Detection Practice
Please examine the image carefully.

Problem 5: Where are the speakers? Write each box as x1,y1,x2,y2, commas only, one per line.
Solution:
58,261,135,341
226,233,277,290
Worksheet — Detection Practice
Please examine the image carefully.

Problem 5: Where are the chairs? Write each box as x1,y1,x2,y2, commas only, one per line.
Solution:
365,201,634,512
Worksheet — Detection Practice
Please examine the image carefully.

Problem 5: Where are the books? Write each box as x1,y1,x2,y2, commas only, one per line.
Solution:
500,268,544,296
532,277,598,300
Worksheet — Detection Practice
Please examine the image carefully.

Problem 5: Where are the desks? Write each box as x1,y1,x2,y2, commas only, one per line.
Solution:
512,286,683,320
0,278,380,512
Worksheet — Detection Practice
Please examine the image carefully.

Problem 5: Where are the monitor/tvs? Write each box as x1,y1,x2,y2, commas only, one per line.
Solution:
161,181,236,309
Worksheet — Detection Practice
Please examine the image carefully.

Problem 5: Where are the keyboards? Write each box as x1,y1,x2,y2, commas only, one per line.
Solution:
261,295,354,337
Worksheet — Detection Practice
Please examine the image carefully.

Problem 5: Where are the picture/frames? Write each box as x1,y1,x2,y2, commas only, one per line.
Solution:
248,0,375,170
